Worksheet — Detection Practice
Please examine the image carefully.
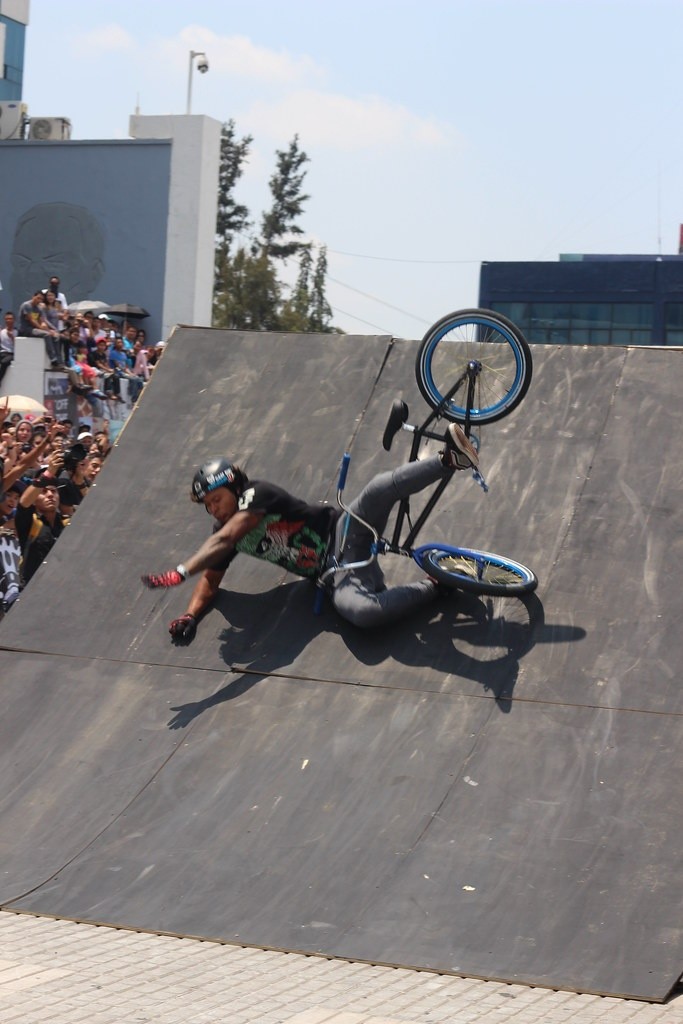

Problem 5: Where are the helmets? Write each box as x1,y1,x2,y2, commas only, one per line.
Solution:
189,458,242,503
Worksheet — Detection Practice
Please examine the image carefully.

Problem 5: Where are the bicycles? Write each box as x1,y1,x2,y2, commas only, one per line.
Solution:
313,307,540,619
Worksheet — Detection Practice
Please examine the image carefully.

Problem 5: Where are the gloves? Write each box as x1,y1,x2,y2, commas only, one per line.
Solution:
140,568,186,589
168,613,199,647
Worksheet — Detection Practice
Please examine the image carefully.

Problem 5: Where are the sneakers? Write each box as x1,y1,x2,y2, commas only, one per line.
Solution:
426,564,477,597
440,423,480,471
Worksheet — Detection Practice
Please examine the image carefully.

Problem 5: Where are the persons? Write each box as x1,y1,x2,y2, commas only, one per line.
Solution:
140,423,481,643
0,274,166,618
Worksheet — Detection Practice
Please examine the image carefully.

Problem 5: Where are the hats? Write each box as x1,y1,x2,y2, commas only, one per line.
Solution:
98,314,111,321
15,419,34,436
154,340,166,351
77,432,93,440
12,478,26,493
79,423,91,431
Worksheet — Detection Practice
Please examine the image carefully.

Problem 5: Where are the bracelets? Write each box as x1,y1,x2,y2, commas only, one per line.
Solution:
175,564,190,582
